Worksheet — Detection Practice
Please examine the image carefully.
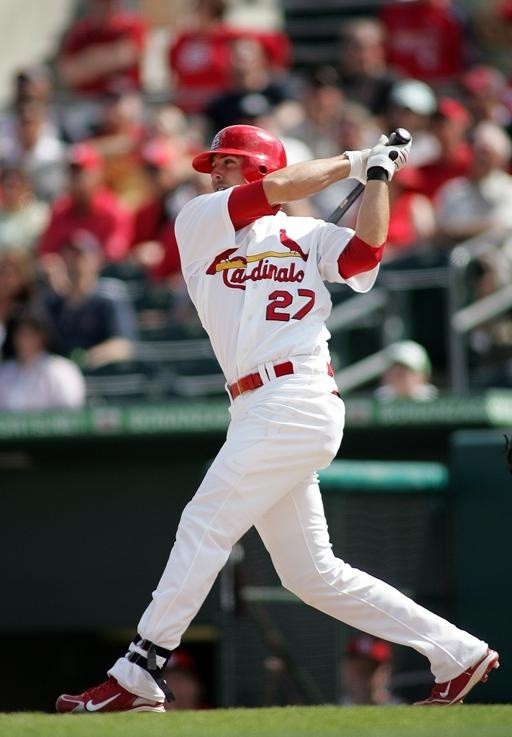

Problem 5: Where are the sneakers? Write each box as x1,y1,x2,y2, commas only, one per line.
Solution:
55,676,166,714
413,648,500,707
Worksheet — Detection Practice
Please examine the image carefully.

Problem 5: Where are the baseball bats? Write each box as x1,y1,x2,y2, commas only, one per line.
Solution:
325,129,412,224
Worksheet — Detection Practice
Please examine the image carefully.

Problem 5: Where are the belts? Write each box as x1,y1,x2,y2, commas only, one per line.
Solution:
227,361,334,403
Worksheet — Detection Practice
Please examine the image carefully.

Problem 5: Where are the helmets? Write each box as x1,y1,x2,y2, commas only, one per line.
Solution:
192,124,287,185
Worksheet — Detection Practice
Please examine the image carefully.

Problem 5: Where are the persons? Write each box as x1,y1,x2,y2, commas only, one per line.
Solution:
338,635,402,706
54,124,503,714
162,652,201,710
0,0,511,409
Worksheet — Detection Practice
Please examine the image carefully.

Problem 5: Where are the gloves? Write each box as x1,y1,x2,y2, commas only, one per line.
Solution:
344,134,413,186
